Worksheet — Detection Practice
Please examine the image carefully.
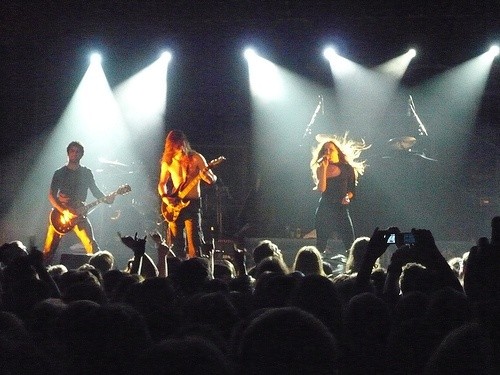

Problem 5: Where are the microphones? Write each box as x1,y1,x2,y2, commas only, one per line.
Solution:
318,157,326,165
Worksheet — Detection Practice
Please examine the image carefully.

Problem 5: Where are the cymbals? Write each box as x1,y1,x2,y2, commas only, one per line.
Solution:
387,137,416,150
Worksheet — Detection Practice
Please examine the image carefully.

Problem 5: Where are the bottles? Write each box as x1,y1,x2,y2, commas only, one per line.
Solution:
296,228,300,238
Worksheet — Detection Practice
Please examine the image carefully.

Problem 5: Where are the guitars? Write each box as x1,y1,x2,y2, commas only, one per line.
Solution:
50,184,132,235
160,155,225,225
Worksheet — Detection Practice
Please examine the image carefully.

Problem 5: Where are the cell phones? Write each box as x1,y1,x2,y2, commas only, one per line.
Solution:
400,233,416,245
384,234,396,243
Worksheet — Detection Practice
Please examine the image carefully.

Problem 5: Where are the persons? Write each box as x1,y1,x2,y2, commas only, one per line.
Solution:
156,129,217,258
0,217,500,375
43,141,115,256
313,141,356,257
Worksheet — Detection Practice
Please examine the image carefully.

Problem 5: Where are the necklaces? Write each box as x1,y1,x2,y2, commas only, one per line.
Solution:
174,157,184,165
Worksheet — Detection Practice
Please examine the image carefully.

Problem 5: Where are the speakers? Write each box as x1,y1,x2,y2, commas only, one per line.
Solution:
60,253,92,270
